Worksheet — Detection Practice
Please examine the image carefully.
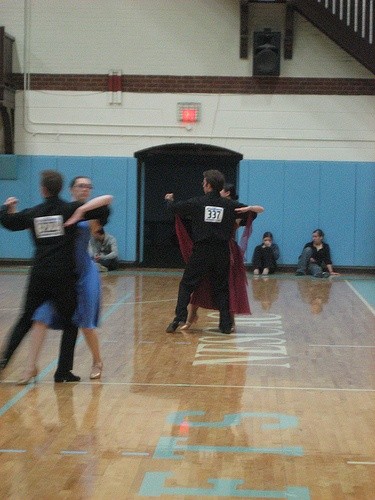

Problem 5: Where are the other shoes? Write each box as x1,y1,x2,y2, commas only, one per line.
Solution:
54,371,80,382
219,324,231,334
167,321,179,332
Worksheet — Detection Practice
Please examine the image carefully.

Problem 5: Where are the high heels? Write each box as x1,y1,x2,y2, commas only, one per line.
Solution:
180,315,198,330
90,361,103,379
15,368,38,385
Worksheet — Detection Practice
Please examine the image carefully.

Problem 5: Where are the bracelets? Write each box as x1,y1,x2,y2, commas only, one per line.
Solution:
248,205,253,211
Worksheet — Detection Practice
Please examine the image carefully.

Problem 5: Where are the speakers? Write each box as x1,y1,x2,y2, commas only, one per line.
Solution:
253,32,281,77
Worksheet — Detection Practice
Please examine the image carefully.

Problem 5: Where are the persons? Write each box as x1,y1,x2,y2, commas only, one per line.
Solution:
5,176,115,387
88,226,121,273
164,168,258,335
0,169,114,385
252,231,280,275
164,181,265,332
293,228,340,278
